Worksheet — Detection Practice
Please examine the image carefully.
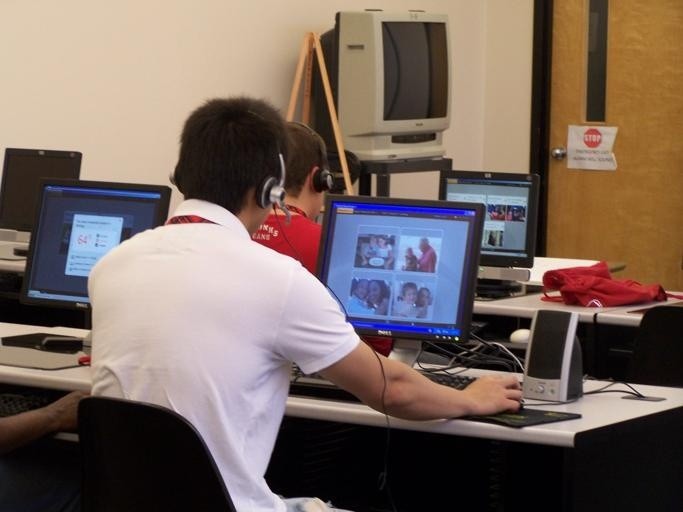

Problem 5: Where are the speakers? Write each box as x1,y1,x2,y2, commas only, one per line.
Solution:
522,309,582,403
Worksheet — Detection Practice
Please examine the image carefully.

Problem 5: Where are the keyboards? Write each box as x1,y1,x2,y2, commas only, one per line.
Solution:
289,373,475,402
0,393,46,418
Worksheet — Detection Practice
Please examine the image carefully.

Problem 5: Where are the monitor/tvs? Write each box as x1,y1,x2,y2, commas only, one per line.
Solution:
0,148,82,256
312,10,451,161
20,178,172,309
321,195,486,369
438,169,540,282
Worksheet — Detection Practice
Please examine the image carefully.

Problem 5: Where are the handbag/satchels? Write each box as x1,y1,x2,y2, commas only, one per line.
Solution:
543,263,667,307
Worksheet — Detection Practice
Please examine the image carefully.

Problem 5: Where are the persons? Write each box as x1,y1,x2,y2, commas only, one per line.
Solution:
1,391,89,455
88,98,523,511
487,205,522,222
253,122,328,270
348,234,435,318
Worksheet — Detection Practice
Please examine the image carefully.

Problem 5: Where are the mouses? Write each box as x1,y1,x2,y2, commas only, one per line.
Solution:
509,328,529,343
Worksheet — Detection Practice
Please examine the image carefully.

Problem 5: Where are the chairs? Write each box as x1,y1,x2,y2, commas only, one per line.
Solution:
77,396,239,511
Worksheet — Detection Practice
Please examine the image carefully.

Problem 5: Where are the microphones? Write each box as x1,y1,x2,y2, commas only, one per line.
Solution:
278,203,291,226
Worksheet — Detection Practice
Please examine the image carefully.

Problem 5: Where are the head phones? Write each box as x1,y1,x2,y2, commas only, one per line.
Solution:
168,110,287,208
288,121,335,193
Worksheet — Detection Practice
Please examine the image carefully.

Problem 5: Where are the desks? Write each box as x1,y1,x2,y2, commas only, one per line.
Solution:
359,157,452,201
0,257,683,449
